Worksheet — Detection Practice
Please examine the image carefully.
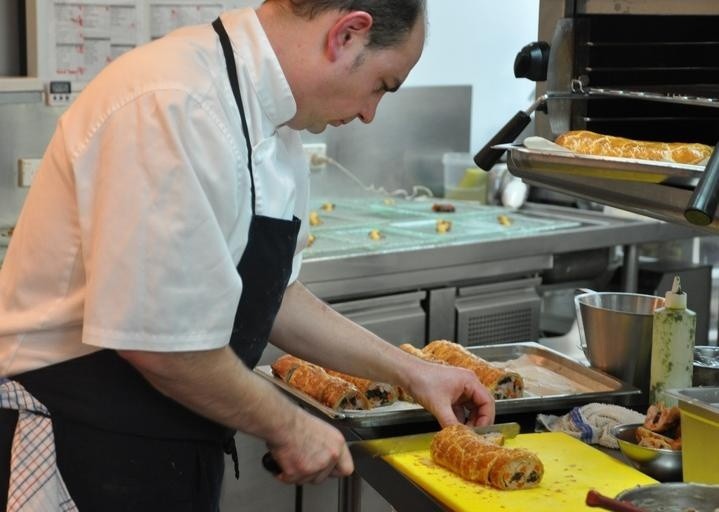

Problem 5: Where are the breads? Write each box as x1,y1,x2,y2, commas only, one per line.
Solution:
430,422,544,491
556,129,713,166
270,339,524,410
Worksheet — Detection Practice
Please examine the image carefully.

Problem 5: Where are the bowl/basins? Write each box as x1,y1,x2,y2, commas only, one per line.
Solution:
607,422,686,484
685,346,719,389
613,482,718,512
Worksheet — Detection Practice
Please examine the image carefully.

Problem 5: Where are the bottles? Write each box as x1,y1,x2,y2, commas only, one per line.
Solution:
651,274,696,404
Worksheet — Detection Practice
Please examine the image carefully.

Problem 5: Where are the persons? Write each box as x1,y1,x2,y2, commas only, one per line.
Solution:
1,0,495,512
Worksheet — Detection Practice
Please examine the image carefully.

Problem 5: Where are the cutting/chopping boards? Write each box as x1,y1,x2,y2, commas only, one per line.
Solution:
360,423,690,511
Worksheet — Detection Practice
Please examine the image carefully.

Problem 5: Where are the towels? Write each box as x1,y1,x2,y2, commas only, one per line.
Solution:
535,402,646,450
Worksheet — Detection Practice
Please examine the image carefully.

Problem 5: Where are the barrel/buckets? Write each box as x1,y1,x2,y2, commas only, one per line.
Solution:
572,292,668,406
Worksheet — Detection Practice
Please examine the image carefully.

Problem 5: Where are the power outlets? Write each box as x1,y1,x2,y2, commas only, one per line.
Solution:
300,143,326,171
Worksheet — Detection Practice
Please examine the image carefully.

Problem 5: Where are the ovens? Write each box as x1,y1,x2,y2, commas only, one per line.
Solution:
474,2,717,233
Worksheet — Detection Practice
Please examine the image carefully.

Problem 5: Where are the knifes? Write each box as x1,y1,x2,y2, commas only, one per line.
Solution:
259,422,522,472
